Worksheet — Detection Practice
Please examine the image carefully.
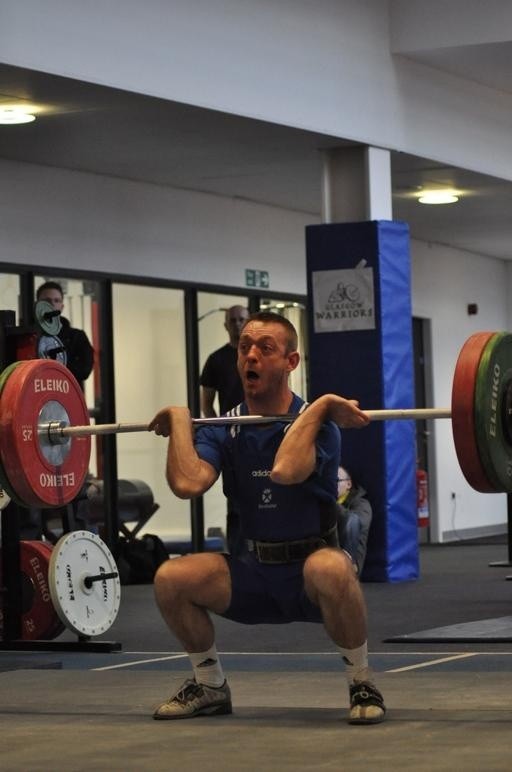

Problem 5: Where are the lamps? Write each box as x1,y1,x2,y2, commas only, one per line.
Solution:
0,113,36,126
418,196,458,204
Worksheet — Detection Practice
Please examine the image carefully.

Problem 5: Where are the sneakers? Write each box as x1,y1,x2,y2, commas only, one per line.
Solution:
348,681,386,724
152,677,232,719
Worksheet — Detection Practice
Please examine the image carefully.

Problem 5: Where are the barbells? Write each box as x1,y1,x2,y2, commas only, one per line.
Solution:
10,299,67,366
12,531,124,640
0,332,512,508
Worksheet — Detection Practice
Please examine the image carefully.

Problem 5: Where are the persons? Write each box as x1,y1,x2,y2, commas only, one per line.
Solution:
334,462,375,585
144,311,387,725
16,280,96,404
197,303,251,419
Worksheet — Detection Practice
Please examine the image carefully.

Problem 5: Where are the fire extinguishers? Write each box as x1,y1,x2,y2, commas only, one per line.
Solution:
416,457,430,528
